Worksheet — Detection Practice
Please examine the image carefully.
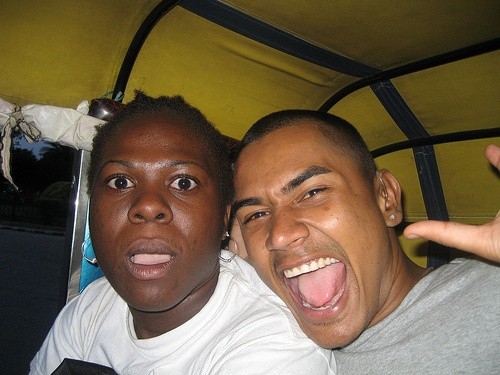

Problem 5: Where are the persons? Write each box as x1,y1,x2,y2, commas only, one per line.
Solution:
26,89,336,375
233,109,500,375
